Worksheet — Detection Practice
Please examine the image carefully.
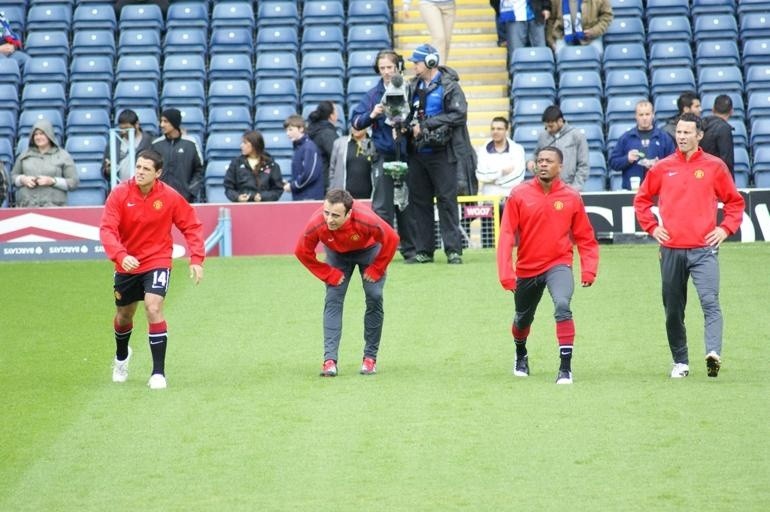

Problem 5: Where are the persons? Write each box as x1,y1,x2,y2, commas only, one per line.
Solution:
631,113,746,380
495,148,601,386
490,1,614,69
296,192,402,378
101,109,205,203
100,146,207,389
10,120,79,207
1,11,21,56
223,131,284,201
350,44,472,265
284,101,376,200
662,93,734,175
476,117,525,247
527,106,590,191
609,101,677,190
419,1,456,64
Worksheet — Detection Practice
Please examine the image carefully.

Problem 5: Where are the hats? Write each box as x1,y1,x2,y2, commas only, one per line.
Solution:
162,109,181,131
407,45,439,63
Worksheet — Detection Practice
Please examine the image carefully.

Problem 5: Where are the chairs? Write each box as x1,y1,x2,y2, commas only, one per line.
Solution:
507,0,770,190
0,1,393,210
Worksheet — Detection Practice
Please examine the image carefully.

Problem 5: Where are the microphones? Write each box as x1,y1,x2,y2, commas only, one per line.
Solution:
416,68,428,76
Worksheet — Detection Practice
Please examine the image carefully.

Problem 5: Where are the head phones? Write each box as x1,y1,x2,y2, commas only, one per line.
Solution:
425,42,437,69
373,51,403,74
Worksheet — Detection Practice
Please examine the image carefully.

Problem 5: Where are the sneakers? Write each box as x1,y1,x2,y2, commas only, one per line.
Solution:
556,370,572,385
113,346,132,382
515,353,529,377
149,373,165,388
448,253,461,263
319,360,339,377
705,351,721,377
671,362,689,377
405,254,432,263
361,359,375,374
